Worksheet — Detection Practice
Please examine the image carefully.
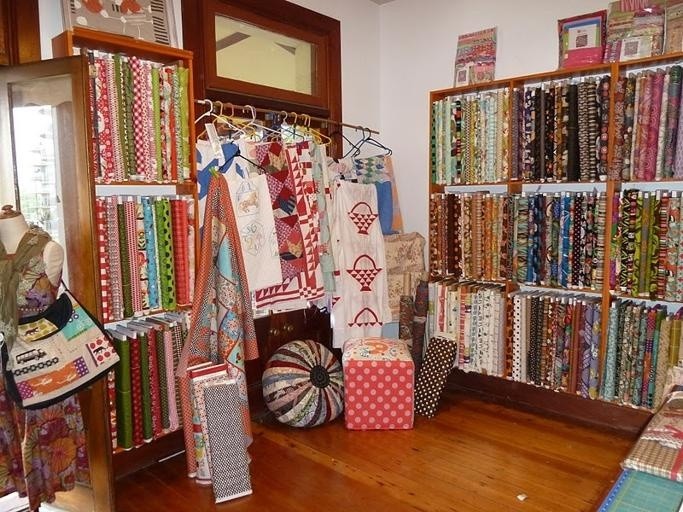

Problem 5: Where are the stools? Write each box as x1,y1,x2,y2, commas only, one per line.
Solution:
262,332,416,436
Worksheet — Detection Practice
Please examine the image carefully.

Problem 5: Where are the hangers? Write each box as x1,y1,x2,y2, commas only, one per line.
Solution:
191,98,392,164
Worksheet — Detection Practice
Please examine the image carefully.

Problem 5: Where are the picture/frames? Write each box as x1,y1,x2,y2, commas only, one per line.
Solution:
63,1,178,47
559,9,607,71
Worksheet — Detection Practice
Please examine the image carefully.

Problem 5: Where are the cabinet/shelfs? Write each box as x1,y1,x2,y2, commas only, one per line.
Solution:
52,25,203,488
421,51,683,430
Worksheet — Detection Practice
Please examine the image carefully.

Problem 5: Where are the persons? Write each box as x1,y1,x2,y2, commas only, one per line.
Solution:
0,205,69,512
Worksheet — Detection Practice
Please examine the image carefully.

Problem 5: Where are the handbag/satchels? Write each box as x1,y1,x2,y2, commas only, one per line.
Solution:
0,276,122,410
